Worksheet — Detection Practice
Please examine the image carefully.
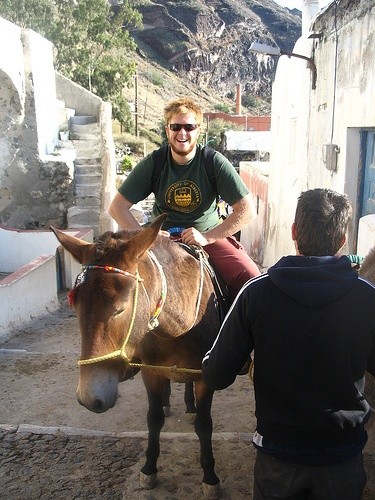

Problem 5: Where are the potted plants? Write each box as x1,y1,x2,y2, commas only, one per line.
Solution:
121,157,132,175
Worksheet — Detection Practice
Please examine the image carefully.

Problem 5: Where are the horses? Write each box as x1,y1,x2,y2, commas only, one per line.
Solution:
49,211,256,500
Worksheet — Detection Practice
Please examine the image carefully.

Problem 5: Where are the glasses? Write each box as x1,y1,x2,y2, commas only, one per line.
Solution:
167,124,200,131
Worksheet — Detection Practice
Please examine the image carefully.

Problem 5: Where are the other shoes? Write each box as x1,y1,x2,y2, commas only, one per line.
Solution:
238,355,252,375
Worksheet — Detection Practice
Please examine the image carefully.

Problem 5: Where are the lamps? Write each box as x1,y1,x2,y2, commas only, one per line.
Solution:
247,43,317,89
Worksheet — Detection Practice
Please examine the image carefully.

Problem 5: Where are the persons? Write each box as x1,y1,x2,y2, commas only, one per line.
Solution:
194,189,375,500
109,100,262,374
